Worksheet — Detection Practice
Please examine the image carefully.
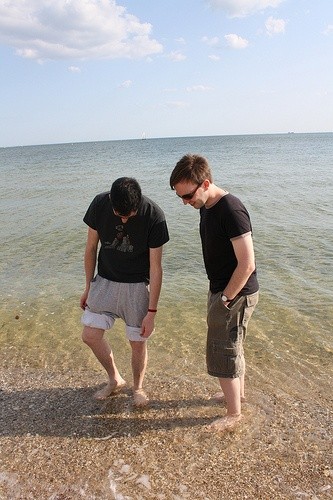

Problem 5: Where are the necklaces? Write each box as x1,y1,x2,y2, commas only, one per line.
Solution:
206,190,226,207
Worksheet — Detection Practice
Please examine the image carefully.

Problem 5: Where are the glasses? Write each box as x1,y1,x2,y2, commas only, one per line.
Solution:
176,182,202,200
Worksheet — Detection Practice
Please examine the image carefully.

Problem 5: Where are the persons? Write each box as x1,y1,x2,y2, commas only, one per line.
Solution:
82,178,173,407
169,155,260,432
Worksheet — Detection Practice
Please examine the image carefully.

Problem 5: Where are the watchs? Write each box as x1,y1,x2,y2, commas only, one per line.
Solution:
221,293,233,302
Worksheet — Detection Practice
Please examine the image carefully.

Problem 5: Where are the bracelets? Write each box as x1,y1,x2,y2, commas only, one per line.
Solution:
148,309,157,312
